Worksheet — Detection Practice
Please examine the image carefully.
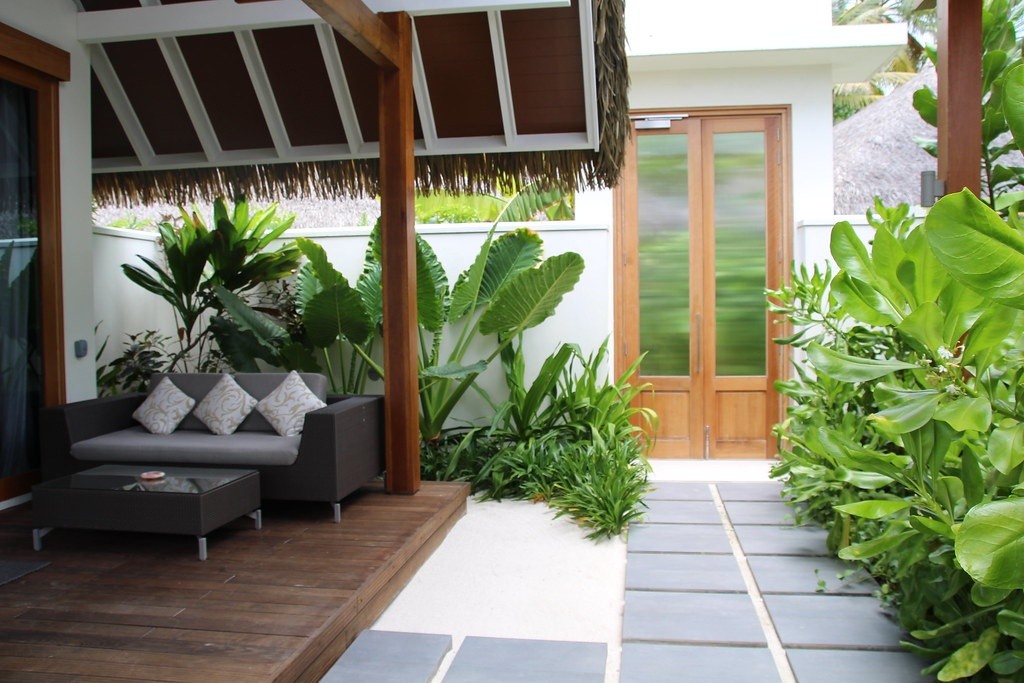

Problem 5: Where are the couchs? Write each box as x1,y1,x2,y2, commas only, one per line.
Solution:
39,372,386,523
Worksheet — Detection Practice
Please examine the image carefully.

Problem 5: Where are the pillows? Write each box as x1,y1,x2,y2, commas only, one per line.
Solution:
192,373,258,438
254,370,328,437
132,375,196,435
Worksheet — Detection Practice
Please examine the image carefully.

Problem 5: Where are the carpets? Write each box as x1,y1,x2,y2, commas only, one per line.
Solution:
0,560,52,586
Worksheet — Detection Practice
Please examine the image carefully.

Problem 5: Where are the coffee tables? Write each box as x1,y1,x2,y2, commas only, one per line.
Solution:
29,464,262,560
